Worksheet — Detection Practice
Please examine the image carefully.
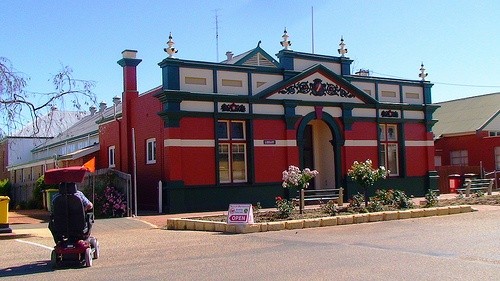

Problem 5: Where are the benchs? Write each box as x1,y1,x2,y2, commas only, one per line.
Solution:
291,187,344,209
456,179,493,196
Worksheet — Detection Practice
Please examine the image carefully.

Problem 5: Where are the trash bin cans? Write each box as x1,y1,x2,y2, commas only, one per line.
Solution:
41,187,60,211
448,172,479,194
0,195,11,224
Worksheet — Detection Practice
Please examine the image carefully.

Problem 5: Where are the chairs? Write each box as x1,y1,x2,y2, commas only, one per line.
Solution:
52,183,88,238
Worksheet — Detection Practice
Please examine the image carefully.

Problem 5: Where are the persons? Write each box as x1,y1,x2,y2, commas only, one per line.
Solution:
50,181,94,231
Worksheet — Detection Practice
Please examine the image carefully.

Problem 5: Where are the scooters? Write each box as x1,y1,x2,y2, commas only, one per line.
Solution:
43,165,100,268
